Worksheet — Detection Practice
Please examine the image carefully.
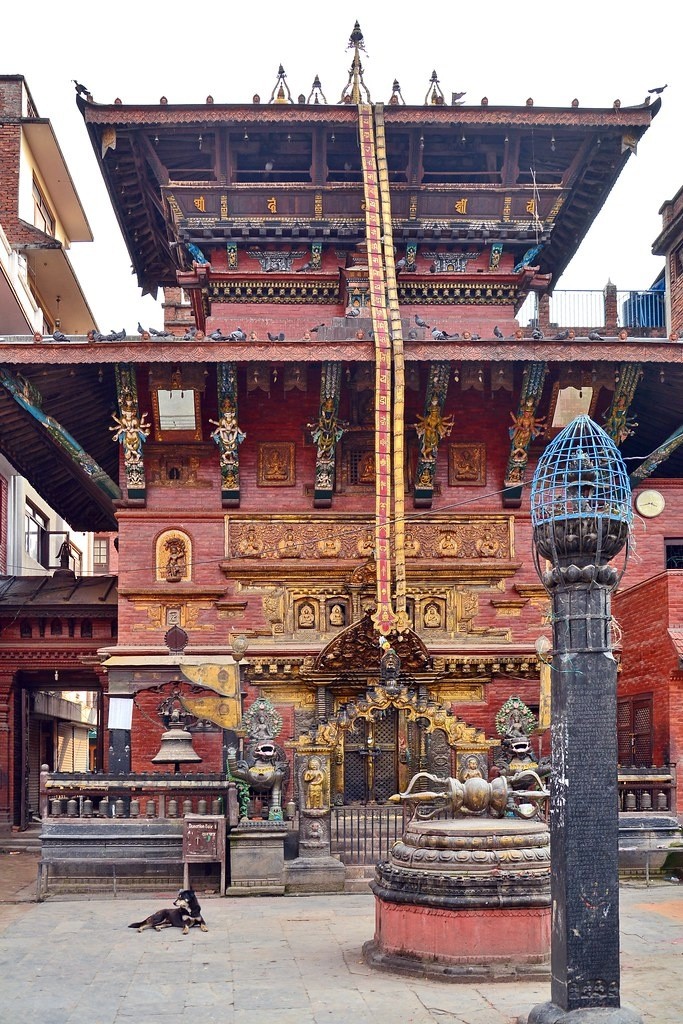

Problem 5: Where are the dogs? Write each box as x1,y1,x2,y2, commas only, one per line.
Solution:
128,890,208,935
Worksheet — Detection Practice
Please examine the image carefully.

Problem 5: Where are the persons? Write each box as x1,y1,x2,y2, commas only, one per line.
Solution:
242,525,499,631
456,452,478,480
462,758,483,784
114,388,632,463
505,709,526,737
264,452,287,476
166,547,184,577
304,758,323,809
252,716,273,738
362,456,375,480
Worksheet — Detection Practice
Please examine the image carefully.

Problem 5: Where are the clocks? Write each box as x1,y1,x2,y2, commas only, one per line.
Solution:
634,490,667,519
